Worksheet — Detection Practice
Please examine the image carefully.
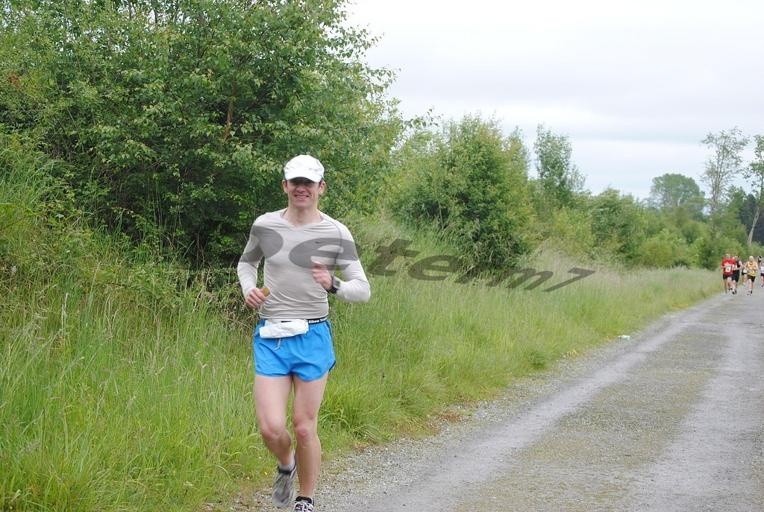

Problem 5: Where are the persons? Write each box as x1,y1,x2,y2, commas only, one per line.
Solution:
236,154,371,512
721,253,763,295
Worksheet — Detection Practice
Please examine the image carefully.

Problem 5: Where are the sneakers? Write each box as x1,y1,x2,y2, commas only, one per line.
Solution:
272,450,297,507
747,291,750,295
728,288,732,291
751,291,752,294
732,290,737,295
293,497,314,512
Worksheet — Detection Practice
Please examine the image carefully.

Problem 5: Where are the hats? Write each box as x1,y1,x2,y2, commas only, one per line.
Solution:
284,154,324,182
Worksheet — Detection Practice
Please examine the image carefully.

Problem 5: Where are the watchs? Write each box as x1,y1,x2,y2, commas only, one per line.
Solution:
326,276,340,297
